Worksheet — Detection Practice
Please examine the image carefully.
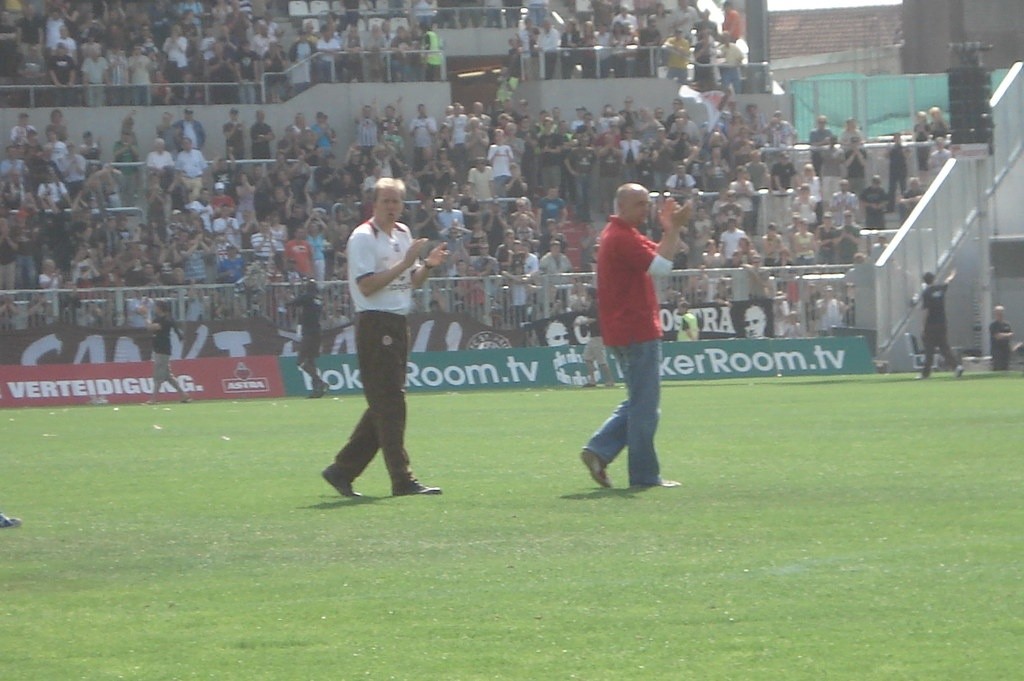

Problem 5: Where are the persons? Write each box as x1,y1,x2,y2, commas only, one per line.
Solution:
488,127,514,208
675,301,700,341
663,26,691,84
135,297,192,404
287,278,329,399
176,136,209,201
581,182,693,487
464,155,499,209
580,286,614,388
917,268,965,379
742,303,768,339
814,284,849,337
828,178,861,226
988,304,1014,372
323,178,443,498
0,0,953,330
884,128,911,202
408,101,438,171
716,29,744,95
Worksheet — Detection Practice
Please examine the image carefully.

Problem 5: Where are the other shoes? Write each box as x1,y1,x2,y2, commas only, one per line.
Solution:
393,479,441,497
0,514,21,528
915,375,928,380
582,450,612,488
322,463,363,497
955,364,963,378
656,479,681,488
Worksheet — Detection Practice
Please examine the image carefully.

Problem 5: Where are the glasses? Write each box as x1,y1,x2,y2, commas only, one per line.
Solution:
621,200,653,208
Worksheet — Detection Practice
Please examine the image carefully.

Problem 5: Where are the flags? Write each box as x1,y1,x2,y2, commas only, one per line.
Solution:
677,81,725,130
